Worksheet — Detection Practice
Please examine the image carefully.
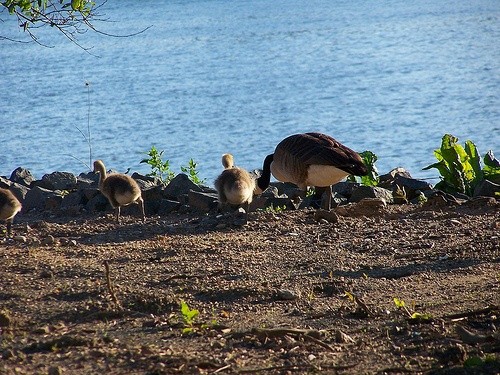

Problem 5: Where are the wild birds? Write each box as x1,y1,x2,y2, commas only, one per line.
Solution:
254,132,368,226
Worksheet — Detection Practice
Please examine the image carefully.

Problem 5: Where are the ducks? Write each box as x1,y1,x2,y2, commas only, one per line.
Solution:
94,160,146,225
215,154,256,225
0,188,22,239
